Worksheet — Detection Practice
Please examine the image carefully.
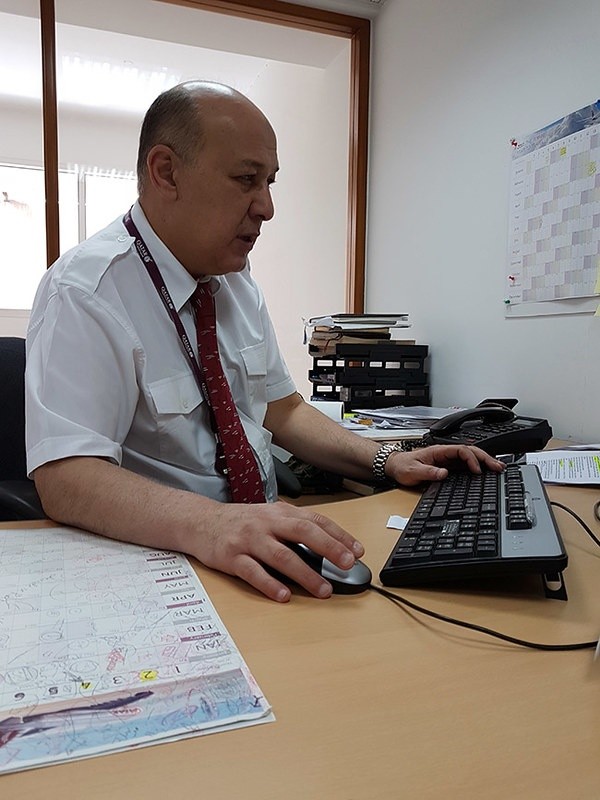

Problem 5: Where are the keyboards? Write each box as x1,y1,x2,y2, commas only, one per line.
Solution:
378,462,569,601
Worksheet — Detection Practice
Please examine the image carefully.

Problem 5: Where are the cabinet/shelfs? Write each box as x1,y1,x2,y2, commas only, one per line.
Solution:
308,344,429,412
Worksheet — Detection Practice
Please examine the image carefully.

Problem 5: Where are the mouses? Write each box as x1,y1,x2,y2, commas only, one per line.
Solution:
272,539,372,595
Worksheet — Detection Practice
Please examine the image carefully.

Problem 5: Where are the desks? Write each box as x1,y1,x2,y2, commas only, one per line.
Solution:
1,437,599,800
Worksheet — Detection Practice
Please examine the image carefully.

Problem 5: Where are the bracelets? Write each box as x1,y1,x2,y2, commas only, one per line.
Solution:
372,441,405,483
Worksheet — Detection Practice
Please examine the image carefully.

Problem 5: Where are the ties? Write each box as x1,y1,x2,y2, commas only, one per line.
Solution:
195,278,266,504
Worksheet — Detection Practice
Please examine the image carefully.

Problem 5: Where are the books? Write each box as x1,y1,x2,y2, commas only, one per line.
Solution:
311,326,414,357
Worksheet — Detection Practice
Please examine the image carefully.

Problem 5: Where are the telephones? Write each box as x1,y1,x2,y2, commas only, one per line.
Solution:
423,408,553,464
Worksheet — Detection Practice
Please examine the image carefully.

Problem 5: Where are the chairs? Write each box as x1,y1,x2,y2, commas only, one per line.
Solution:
0,336,303,522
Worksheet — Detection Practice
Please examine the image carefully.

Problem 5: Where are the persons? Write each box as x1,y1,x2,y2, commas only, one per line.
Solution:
23,78,507,608
